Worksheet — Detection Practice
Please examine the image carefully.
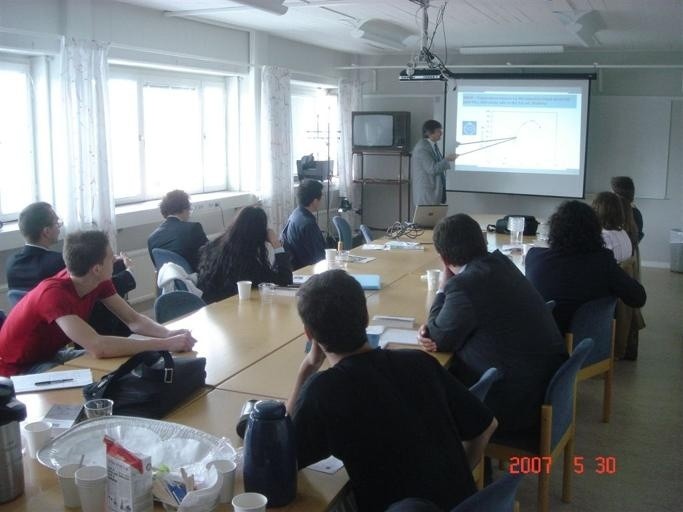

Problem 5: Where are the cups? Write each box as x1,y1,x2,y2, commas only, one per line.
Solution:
237,281,253,300
231,491,269,512
206,460,237,504
426,269,440,290
23,420,53,460
507,216,525,245
55,461,84,509
258,283,274,305
337,250,351,268
324,248,337,263
73,463,108,512
84,398,114,421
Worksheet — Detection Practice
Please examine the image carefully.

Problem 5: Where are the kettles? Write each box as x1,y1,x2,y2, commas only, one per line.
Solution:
0,374,28,503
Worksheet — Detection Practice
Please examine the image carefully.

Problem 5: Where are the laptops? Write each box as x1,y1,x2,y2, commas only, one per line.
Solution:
405,205,449,229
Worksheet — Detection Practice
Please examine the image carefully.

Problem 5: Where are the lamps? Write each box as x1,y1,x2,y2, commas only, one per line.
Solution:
565,9,606,50
352,17,420,54
458,42,564,58
229,1,289,16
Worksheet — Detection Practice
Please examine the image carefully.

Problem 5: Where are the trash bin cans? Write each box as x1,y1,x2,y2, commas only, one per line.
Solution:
669,228,683,273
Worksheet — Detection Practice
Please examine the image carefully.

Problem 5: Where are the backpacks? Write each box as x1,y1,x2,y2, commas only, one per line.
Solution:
487,214,540,237
80,348,205,420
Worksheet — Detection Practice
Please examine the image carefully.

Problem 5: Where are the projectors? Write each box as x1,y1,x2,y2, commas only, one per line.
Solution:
399,69,450,81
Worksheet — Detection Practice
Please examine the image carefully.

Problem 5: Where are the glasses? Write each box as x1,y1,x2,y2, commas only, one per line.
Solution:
41,219,64,228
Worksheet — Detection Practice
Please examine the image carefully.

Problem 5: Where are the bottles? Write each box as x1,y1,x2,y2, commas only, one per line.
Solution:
236,400,260,439
242,398,300,509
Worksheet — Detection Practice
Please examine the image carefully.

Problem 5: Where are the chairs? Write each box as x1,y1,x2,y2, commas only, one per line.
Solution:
618,231,643,282
560,293,620,424
474,330,604,511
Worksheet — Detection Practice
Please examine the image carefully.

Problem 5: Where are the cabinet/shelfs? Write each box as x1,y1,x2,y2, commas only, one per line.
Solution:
348,149,412,235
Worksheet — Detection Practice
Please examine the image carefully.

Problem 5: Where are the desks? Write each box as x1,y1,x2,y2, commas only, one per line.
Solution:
0,213,551,512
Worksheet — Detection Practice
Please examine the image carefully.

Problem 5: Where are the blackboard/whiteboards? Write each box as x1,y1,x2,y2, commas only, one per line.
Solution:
424,93,674,203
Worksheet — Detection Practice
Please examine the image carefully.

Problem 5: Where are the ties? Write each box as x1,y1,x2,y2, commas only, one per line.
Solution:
434,144,441,162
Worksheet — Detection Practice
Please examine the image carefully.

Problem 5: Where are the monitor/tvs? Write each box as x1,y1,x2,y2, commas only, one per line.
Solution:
352,111,410,152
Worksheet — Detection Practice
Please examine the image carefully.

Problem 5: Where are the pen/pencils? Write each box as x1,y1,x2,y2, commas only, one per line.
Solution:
33,378,78,388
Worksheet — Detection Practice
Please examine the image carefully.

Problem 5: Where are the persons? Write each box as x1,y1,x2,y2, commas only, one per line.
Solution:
410,119,456,206
